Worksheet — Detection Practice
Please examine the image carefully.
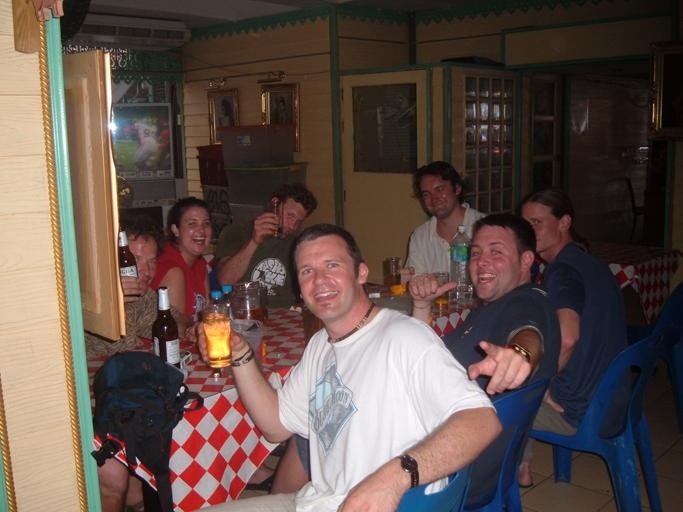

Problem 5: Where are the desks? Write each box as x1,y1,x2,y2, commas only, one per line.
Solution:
86,242,683,512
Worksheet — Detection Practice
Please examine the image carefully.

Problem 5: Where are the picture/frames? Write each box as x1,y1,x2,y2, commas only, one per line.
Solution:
260,82,300,152
113,102,174,179
207,88,240,145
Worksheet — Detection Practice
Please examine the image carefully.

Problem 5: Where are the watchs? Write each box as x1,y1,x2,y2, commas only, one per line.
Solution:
395,455,419,488
504,342,536,368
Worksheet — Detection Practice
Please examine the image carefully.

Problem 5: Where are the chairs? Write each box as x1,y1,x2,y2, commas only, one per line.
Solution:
463,378,547,512
396,464,473,512
528,334,665,512
650,280,683,433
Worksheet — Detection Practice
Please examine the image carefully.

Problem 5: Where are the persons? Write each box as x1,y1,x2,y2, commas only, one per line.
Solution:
96,455,146,511
266,209,562,494
195,222,504,511
83,213,200,359
149,193,277,492
123,113,170,171
213,181,320,456
515,188,651,488
399,158,489,285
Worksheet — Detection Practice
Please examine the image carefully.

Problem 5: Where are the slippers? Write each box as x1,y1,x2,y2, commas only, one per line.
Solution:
246,471,275,490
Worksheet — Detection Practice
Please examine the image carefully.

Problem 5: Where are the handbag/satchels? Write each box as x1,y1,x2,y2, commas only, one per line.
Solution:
92,352,190,472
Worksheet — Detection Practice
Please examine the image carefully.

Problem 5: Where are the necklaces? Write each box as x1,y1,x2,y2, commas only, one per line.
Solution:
326,302,376,346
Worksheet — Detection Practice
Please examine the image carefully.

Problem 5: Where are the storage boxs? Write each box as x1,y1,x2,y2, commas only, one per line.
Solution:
197,126,309,239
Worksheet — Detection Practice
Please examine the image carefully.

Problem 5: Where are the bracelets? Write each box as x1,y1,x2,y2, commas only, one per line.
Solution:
230,346,255,368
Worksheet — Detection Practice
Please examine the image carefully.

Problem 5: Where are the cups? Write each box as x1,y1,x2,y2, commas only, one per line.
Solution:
202,304,233,369
265,202,284,237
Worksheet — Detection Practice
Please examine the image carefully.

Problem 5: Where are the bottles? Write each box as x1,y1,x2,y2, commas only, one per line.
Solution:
222,285,233,302
152,287,180,369
118,230,140,303
450,226,472,283
209,291,231,313
